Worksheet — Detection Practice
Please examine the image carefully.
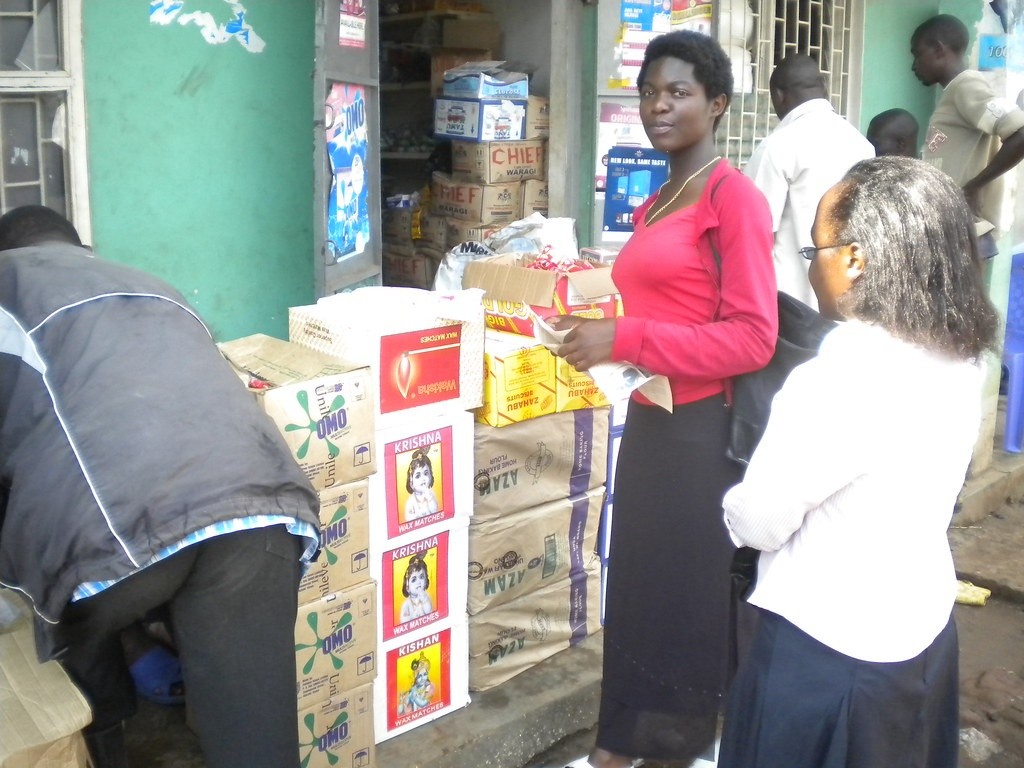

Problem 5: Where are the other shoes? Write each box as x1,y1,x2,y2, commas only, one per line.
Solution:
562,755,645,768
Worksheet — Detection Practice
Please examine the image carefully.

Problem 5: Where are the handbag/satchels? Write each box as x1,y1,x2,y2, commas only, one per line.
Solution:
705,173,839,466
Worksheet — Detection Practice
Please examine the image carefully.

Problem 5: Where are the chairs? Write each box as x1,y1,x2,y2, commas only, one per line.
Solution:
1000,253,1023,453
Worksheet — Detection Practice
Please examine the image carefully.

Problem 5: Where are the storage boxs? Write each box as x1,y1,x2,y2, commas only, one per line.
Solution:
0,0,622,768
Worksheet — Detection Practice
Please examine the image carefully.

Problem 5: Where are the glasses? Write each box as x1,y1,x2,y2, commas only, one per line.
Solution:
799,243,851,261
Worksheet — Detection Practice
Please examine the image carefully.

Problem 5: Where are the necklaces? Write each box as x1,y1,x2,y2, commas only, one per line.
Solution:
645,155,721,226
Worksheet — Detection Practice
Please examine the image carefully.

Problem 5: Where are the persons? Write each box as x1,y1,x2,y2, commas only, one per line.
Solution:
719,55,878,718
0,208,322,768
911,15,1024,513
541,31,779,768
867,108,919,159
719,157,1001,768
392,447,439,714
